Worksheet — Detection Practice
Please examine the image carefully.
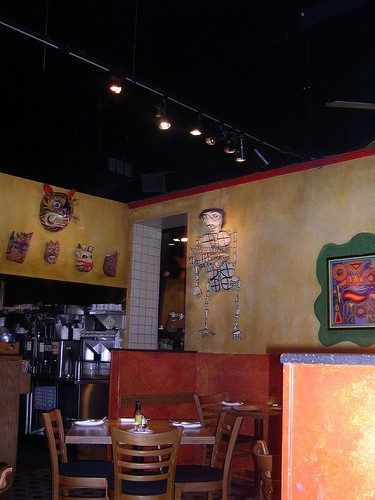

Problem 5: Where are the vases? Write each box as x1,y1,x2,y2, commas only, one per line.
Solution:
166,317,178,333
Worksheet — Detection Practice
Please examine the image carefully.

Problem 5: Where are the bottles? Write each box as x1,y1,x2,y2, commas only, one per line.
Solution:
135,401,142,423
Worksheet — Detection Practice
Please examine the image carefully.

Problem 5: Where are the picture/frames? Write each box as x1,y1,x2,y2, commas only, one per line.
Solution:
326,252,375,330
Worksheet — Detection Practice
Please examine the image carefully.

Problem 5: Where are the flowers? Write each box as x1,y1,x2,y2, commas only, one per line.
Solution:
169,311,185,320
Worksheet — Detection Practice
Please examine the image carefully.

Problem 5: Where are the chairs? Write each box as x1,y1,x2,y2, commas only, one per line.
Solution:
41,384,282,500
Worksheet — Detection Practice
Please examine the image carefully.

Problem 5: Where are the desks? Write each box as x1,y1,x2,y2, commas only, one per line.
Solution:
64,419,216,473
205,400,282,488
158,328,183,352
